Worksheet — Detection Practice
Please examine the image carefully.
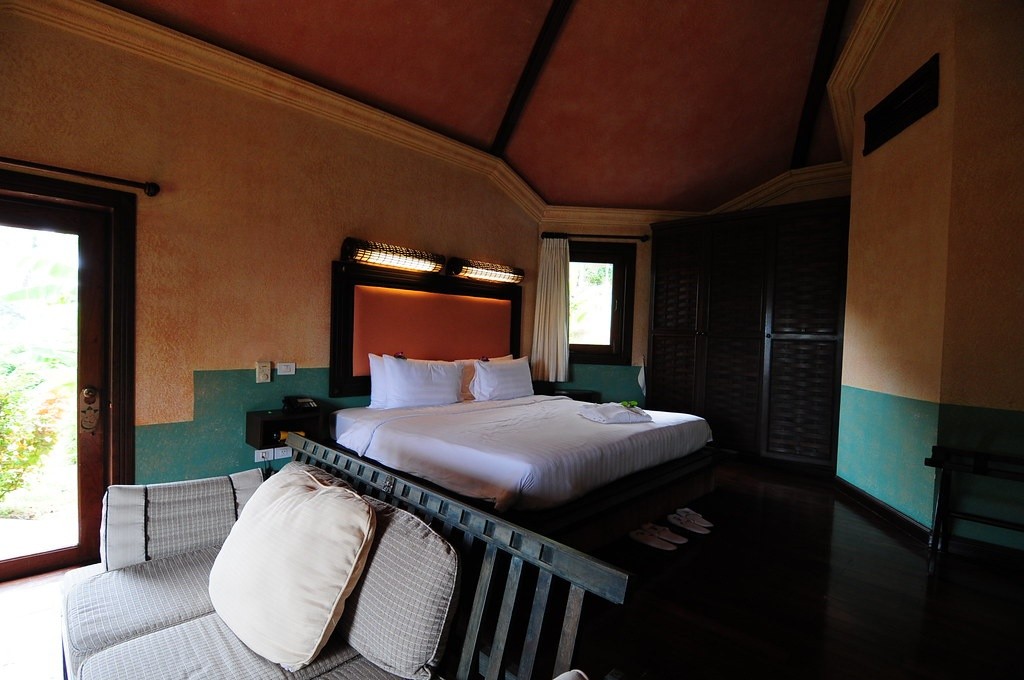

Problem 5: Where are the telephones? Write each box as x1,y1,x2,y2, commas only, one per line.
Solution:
282,396,321,413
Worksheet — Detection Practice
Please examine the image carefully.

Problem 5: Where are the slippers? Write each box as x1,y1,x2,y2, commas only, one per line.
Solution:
630,522,688,550
667,507,713,534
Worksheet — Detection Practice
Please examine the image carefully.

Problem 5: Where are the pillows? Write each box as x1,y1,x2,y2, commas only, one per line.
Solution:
367,352,384,410
468,356,534,402
454,355,514,399
382,354,465,407
208,461,459,680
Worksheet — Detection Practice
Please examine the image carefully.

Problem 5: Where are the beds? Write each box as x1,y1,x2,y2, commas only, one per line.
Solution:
328,260,722,553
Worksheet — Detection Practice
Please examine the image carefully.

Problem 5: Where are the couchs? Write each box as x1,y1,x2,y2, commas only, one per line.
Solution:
61,433,631,680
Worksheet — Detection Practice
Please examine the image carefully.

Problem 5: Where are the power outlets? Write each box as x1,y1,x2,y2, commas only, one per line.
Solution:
254,449,273,463
275,446,293,460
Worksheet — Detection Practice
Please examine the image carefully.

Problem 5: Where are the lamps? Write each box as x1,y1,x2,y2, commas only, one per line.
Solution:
342,237,446,274
449,256,525,285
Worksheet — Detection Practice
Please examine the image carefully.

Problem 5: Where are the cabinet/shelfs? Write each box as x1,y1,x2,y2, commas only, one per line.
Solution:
647,194,851,479
246,412,322,450
